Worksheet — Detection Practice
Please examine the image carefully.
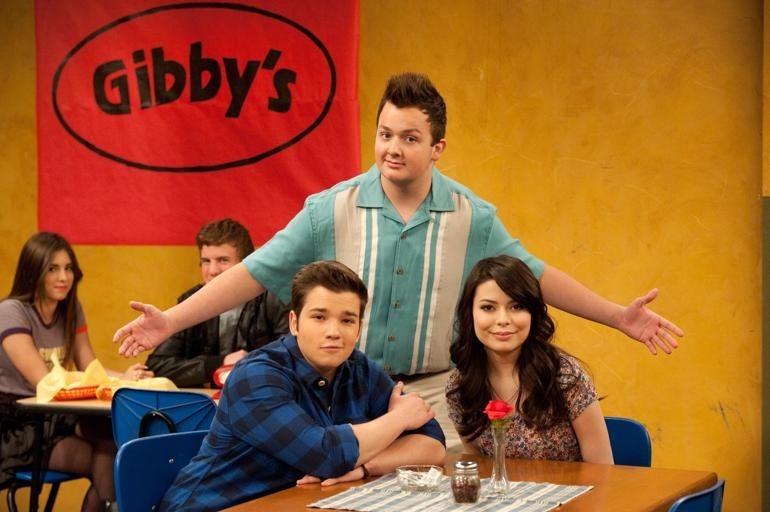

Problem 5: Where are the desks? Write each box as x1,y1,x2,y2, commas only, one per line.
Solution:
223,450,718,510
14,388,221,511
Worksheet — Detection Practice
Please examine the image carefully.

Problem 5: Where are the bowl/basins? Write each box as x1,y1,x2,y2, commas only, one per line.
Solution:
395,464,444,497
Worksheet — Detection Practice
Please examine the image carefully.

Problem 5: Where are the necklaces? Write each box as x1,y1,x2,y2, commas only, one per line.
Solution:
487,379,520,402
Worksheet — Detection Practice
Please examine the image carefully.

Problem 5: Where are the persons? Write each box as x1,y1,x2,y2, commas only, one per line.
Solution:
109,72,686,450
159,259,447,512
0,232,155,512
144,216,289,387
447,254,614,465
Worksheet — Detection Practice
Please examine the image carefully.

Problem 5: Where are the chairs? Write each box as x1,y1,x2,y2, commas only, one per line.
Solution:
109,389,218,512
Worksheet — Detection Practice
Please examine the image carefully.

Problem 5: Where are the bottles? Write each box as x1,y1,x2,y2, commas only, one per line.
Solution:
451,460,481,505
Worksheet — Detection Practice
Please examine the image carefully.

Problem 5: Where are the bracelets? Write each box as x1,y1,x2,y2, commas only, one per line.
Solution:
360,462,370,479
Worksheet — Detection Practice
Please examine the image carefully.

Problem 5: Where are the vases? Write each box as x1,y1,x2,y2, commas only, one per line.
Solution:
488,426,512,493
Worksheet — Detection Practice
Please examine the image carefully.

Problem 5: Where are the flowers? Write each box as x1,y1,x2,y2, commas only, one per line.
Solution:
482,398,513,430
